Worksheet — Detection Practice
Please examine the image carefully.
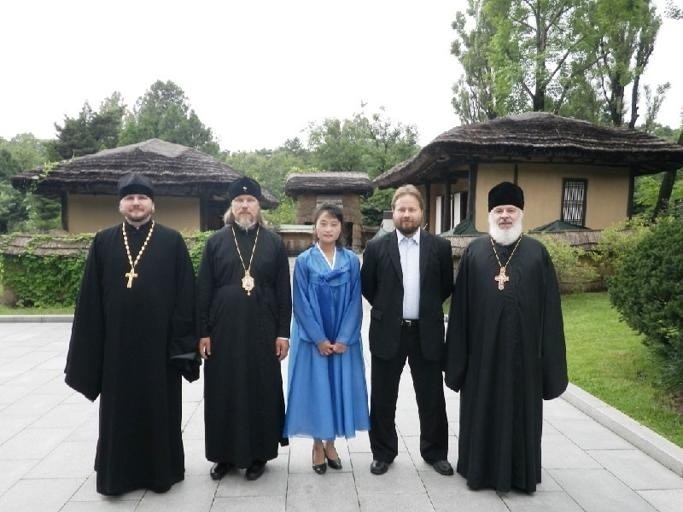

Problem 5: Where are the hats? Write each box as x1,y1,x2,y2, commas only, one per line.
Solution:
486,182,525,212
117,173,155,200
227,176,262,201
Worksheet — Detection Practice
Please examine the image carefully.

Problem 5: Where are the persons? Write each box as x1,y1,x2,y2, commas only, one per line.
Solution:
361,183,455,477
190,176,292,479
65,168,202,495
443,179,568,494
282,201,369,478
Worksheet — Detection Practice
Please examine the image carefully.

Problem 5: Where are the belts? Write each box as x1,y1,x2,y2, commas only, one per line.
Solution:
399,318,419,328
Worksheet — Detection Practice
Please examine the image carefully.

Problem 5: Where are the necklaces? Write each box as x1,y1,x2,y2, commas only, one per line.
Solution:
121,219,156,289
230,225,259,296
489,233,524,292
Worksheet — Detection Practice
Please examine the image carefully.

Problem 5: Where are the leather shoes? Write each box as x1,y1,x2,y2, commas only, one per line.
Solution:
421,452,455,476
311,444,327,476
322,441,344,472
243,460,267,480
369,457,390,475
208,459,237,480
